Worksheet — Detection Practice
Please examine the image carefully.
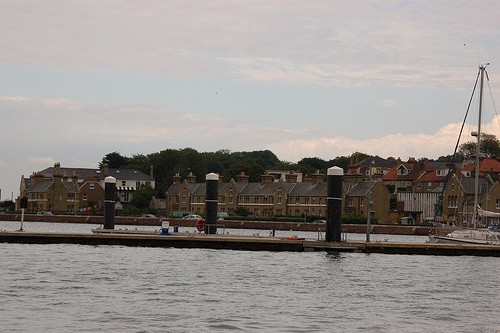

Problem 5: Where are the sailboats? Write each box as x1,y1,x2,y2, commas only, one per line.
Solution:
430,62,499,247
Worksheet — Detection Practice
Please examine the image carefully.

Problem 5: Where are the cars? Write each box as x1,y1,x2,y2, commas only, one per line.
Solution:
183,214,202,220
217,212,229,219
314,219,326,223
142,214,156,218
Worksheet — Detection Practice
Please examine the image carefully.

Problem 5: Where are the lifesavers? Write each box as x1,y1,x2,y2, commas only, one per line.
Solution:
195,219,206,231
